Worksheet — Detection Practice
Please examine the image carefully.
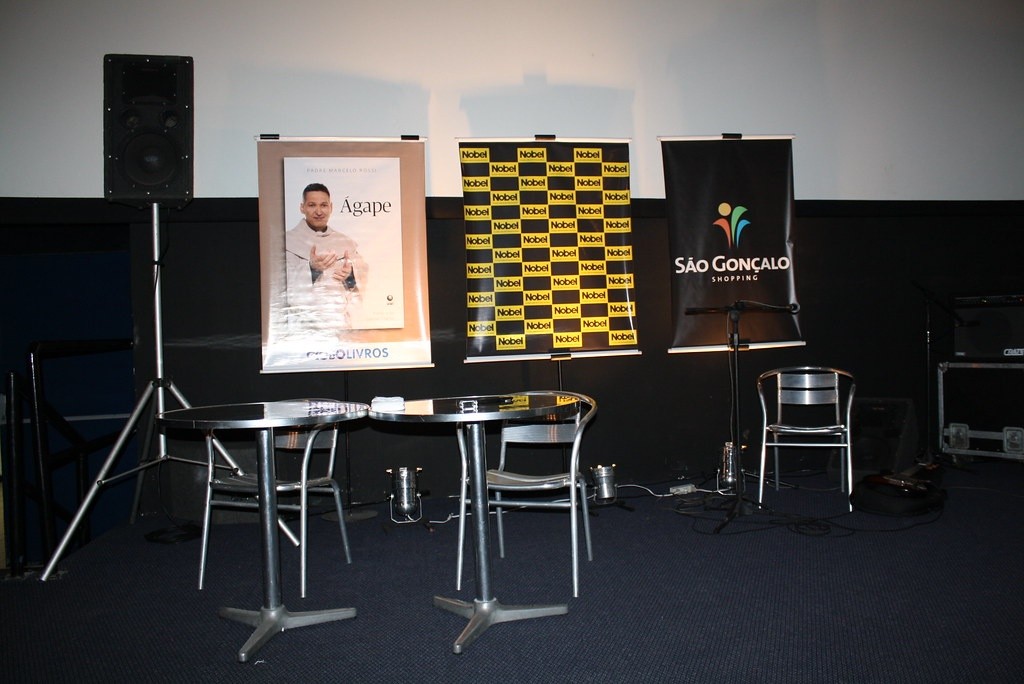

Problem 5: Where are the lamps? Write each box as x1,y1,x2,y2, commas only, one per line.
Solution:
716,443,748,497
384,465,424,524
588,464,618,504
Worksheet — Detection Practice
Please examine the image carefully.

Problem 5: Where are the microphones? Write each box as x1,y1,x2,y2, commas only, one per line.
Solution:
787,301,800,313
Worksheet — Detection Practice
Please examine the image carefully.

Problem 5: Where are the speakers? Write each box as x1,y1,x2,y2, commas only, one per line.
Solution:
951,294,1023,362
838,394,918,478
100,53,194,201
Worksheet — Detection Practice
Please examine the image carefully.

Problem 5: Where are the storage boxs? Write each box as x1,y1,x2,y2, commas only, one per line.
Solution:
939,294,1024,466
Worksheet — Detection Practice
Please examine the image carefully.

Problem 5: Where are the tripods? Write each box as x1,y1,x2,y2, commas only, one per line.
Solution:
35,201,299,585
682,300,790,533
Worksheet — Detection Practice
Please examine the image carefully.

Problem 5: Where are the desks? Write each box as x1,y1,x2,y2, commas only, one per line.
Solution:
158,401,369,663
368,395,581,655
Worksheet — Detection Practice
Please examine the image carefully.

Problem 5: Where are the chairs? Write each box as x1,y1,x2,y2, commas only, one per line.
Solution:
196,398,354,599
755,366,857,512
455,390,597,591
